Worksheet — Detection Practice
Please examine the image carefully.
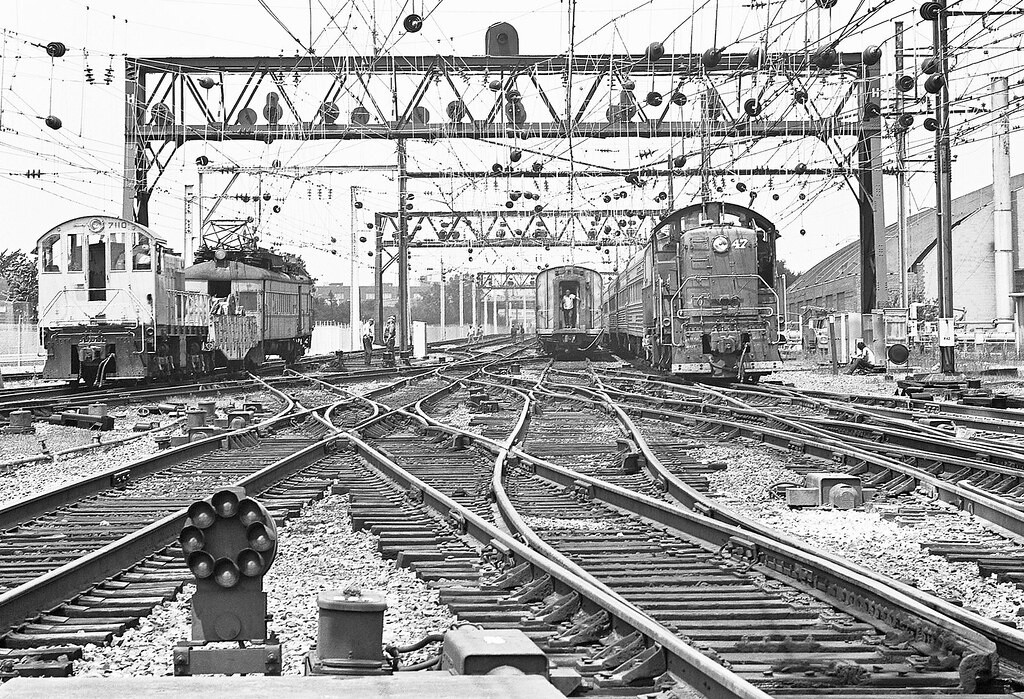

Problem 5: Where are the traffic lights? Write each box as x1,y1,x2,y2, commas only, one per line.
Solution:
179,485,278,645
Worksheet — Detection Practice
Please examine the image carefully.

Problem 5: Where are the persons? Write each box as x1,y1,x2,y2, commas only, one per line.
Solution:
519,325,524,344
362,319,374,366
511,324,519,343
561,290,581,328
467,324,484,345
843,342,875,375
383,315,396,361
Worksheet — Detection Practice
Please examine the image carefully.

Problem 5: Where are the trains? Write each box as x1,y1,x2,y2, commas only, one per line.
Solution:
602,200,785,383
534,265,603,361
30,215,318,387
